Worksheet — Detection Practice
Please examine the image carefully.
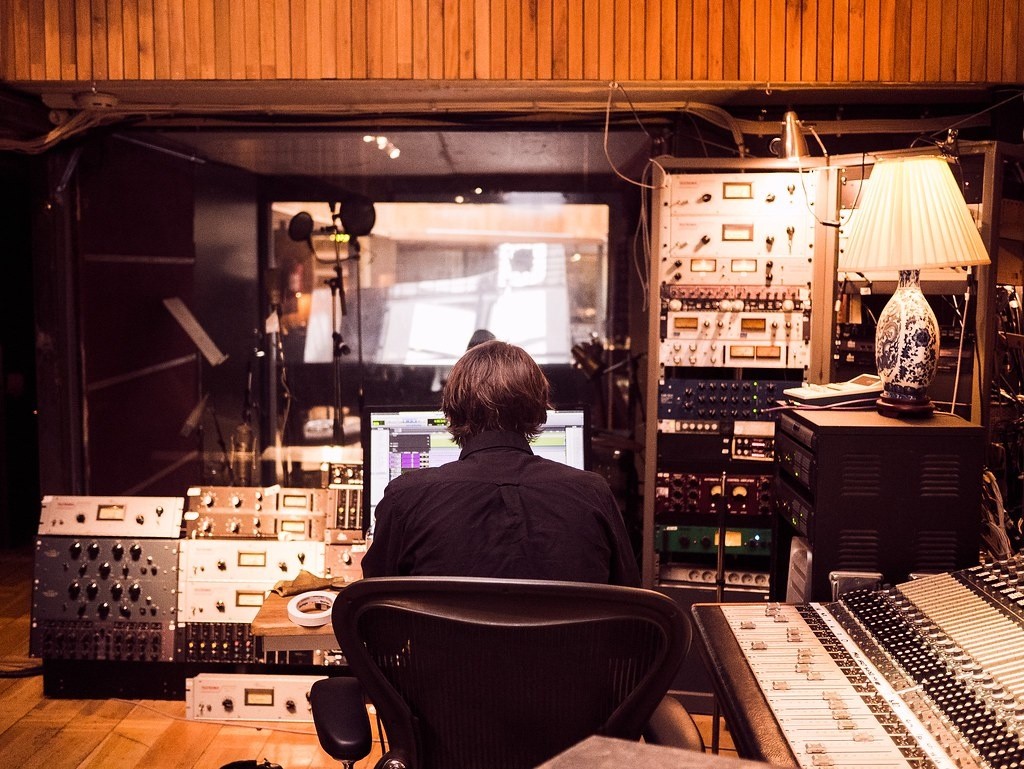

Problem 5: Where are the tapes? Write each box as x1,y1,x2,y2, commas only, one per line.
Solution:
287,591,337,627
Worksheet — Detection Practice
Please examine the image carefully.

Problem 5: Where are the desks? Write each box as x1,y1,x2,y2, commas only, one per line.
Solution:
251,579,341,652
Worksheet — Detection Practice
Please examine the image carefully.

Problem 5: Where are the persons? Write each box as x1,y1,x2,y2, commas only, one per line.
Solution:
361,341,642,590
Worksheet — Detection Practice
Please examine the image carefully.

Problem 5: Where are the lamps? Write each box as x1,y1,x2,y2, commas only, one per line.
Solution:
838,153,993,419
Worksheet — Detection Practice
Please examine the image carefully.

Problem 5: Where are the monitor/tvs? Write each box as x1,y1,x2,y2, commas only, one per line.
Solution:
361,405,592,542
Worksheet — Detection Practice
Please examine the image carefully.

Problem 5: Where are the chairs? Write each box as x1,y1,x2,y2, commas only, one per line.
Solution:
313,575,707,769
